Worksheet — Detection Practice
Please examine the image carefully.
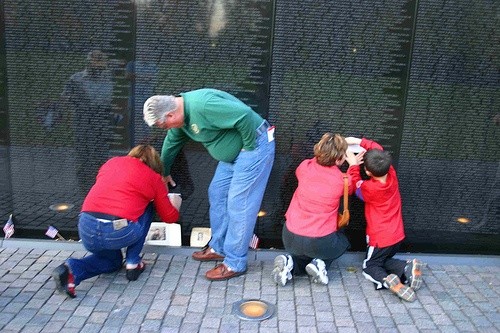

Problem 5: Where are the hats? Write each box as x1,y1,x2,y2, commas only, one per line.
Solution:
87,50,107,69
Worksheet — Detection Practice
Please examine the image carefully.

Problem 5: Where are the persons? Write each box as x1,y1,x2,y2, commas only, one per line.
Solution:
54,143,182,298
141,89,276,281
345,136,423,302
196,232,204,241
271,133,365,287
59,49,115,196
150,228,160,240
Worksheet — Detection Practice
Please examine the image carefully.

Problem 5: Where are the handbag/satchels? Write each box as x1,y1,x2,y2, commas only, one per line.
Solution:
336,172,350,228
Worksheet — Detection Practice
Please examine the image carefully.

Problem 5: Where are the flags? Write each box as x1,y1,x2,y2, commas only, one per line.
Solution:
44,226,58,238
2,219,15,239
249,234,259,250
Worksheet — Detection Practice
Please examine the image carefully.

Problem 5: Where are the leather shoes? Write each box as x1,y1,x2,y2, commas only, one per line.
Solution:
206,262,247,280
192,244,225,260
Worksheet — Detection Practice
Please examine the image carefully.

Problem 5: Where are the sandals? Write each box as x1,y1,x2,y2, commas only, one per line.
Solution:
126,260,145,281
53,263,77,298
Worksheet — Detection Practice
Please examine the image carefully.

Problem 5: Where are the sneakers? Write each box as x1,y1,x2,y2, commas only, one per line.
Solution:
386,273,417,301
271,253,294,286
405,257,422,290
306,258,329,284
